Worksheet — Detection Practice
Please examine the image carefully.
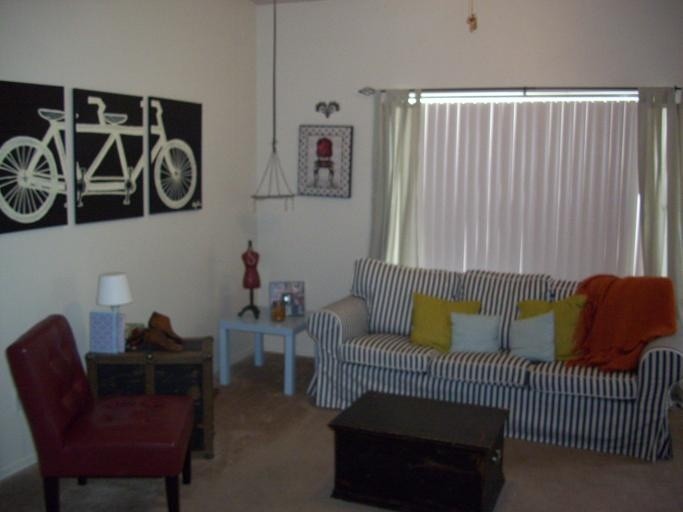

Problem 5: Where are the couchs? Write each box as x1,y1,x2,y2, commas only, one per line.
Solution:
307,257,683,463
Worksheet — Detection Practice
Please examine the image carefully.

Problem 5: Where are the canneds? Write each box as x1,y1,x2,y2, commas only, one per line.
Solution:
271,301,288,322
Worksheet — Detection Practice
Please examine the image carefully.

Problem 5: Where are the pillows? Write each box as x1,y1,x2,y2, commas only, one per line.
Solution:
449,312,500,354
518,295,588,361
509,309,555,362
411,293,481,353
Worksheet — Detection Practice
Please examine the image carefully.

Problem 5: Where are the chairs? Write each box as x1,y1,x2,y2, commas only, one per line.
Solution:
5,314,193,512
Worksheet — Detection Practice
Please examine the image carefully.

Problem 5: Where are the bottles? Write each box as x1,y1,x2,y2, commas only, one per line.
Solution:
271,300,285,322
282,294,294,321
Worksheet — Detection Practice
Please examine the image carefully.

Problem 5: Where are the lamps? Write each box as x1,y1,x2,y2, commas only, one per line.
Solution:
96,273,134,352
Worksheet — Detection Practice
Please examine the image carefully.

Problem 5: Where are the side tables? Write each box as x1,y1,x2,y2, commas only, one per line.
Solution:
85,335,214,460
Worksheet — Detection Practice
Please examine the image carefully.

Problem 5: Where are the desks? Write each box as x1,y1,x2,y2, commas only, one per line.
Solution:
218,307,310,397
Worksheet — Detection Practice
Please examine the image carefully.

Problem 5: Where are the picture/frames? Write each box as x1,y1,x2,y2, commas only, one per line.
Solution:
297,125,352,198
